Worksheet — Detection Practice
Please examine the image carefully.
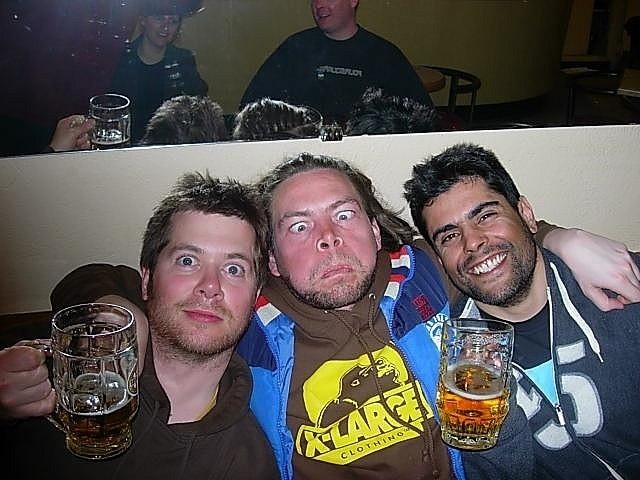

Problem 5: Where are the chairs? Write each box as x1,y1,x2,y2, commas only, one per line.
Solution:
564,71,640,124
413,63,481,127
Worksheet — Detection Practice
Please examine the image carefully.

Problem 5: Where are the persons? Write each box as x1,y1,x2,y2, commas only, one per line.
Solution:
0,155,640,480
343,87,453,137
27,93,231,155
406,140,640,479
0,170,280,479
238,0,435,121
234,97,320,140
111,0,210,143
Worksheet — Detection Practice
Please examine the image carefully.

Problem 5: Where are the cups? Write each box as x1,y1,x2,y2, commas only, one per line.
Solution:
70,93,132,149
23,303,140,459
435,318,515,450
